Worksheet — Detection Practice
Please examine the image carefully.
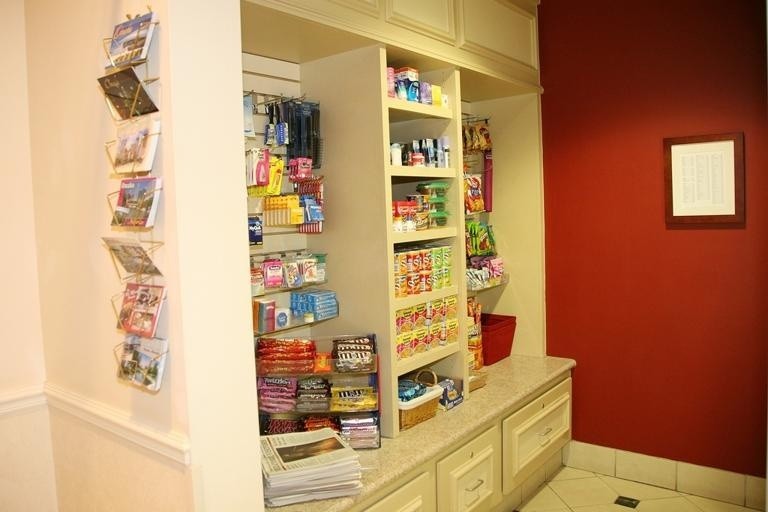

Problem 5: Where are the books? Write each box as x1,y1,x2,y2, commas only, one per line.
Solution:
97,11,168,394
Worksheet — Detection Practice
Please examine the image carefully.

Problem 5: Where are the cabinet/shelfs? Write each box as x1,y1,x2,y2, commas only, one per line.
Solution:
239,1,576,512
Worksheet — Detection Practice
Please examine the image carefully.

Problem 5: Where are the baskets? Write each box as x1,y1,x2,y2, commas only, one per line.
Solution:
399,369,444,430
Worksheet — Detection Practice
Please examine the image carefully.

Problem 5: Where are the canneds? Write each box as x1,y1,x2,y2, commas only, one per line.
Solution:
394,243,452,299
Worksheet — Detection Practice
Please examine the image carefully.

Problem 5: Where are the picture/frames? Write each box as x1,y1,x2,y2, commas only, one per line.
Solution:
663,131,746,226
